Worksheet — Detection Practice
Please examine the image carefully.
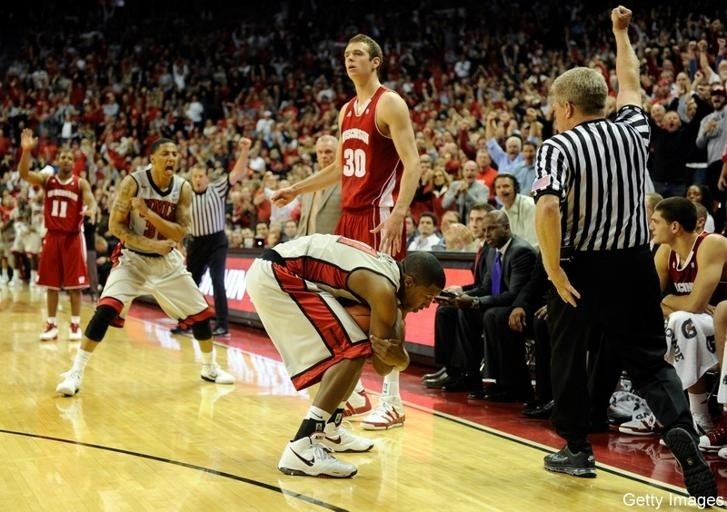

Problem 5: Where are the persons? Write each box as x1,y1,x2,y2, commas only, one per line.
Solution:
372,1,726,468
55,137,237,396
244,232,447,478
1,2,372,301
529,6,720,510
167,135,252,340
258,34,421,430
18,128,98,341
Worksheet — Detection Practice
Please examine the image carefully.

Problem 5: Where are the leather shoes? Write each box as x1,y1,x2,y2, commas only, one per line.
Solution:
419,365,556,416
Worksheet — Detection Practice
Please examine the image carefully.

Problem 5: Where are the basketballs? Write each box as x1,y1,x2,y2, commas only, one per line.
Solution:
345,303,370,335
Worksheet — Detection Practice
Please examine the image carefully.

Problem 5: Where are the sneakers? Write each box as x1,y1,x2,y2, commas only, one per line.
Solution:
597,376,727,459
170,325,192,335
67,321,81,341
211,326,231,338
56,367,82,396
39,320,60,341
664,426,719,507
363,401,407,431
201,365,234,386
276,432,358,478
334,391,372,417
311,422,375,453
542,445,598,477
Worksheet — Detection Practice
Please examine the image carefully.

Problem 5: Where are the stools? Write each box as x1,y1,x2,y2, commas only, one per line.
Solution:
702,369,725,422
